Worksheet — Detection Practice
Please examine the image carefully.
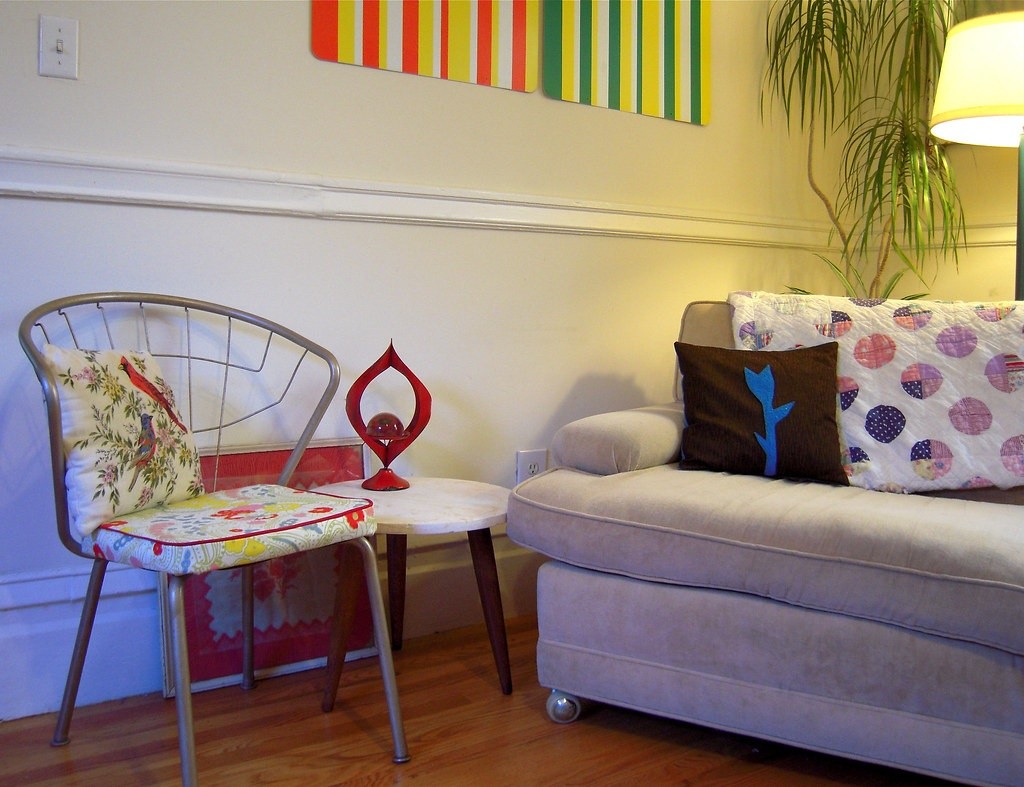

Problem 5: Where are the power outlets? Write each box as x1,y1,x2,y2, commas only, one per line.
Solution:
515,450,548,486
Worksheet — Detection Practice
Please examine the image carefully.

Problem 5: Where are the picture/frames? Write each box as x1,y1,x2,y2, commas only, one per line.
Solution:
162,438,381,699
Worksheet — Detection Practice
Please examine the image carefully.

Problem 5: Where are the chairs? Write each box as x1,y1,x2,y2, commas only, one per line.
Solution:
19,292,412,787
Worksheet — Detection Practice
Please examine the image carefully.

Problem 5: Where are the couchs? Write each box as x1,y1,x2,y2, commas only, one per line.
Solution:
507,291,1023,787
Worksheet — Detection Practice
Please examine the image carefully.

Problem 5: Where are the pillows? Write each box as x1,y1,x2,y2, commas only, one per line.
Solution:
674,342,851,487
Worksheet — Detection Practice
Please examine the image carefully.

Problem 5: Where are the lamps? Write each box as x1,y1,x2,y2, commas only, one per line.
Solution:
929,11,1024,302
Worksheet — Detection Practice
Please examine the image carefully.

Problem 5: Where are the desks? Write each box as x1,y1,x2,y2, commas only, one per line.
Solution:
311,476,515,714
